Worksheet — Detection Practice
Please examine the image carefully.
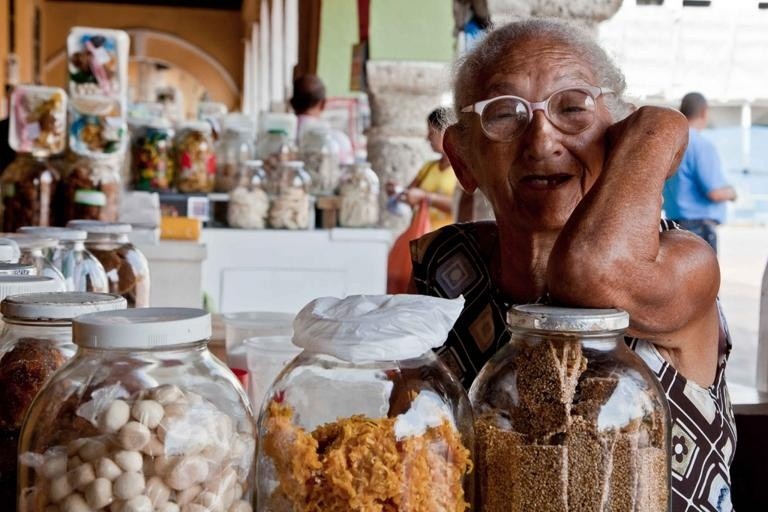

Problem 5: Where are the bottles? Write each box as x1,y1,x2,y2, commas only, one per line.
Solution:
469,302,672,511
1,287,304,512
0,110,381,228
0,214,149,309
253,295,479,511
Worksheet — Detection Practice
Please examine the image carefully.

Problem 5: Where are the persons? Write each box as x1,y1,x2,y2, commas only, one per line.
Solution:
658,90,738,257
397,105,460,235
287,74,354,170
385,16,741,512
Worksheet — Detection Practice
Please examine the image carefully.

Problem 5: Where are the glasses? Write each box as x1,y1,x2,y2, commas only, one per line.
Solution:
457,86,617,136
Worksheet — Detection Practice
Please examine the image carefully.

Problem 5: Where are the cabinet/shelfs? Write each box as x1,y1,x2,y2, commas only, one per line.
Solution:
198,226,392,322
125,237,209,309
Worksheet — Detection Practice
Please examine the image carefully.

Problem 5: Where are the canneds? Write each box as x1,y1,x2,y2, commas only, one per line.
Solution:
16,294,671,511
1,220,150,512
1,117,382,232
